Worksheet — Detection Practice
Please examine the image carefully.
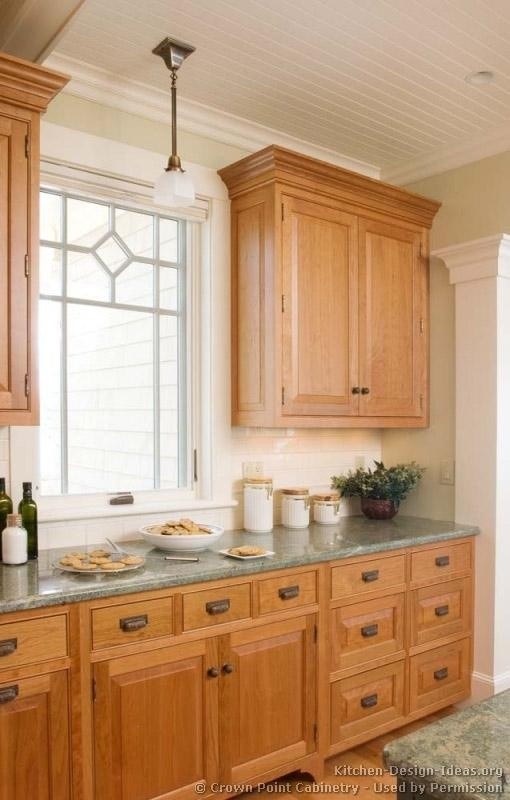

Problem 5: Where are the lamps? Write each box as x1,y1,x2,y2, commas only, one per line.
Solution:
149,35,197,208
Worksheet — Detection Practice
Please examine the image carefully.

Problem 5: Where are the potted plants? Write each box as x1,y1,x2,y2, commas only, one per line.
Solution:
329,457,428,519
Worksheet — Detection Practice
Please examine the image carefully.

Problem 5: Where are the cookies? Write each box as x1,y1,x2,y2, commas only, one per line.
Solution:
147,517,211,537
224,544,267,558
59,547,144,571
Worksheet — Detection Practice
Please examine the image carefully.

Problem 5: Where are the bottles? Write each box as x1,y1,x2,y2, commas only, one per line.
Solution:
314,492,341,524
17,482,38,559
2,514,28,565
0,478,13,561
243,476,274,532
282,486,309,529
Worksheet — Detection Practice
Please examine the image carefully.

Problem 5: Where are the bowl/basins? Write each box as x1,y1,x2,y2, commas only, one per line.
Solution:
139,523,225,555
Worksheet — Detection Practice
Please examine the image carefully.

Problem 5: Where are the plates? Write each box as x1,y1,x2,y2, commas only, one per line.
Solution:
218,548,275,560
52,552,147,576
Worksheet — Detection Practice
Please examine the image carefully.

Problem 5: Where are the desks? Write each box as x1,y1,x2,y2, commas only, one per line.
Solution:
381,685,510,799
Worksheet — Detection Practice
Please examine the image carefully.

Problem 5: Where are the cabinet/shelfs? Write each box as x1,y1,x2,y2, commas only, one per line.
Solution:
1,52,70,427
217,143,443,427
324,529,478,760
79,558,324,800
0,598,79,800
